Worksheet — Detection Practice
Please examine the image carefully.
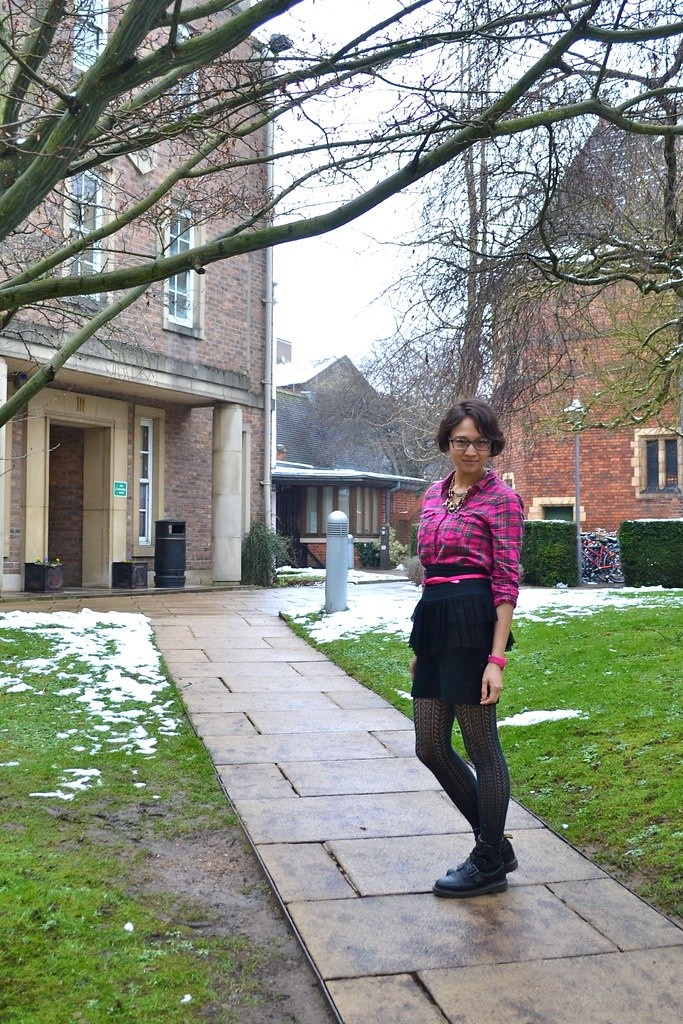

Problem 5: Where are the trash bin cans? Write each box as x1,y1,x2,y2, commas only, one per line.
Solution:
153,519,187,587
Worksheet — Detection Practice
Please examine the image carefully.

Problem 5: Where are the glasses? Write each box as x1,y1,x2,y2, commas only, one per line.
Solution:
446,434,493,451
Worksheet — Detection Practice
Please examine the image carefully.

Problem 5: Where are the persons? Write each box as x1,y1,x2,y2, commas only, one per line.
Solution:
408,399,525,899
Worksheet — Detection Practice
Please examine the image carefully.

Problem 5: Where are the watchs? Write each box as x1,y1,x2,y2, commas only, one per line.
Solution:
488,654,507,670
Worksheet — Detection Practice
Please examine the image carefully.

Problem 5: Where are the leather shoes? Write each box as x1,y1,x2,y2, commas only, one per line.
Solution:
446,832,519,880
433,860,509,899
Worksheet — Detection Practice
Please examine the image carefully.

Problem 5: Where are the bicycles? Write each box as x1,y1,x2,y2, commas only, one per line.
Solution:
579,528,625,584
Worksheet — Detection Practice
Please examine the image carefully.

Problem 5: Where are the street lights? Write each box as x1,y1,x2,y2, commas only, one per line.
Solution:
563,397,587,584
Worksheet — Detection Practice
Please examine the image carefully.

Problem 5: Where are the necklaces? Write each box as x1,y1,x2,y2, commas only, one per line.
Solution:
447,468,487,515
